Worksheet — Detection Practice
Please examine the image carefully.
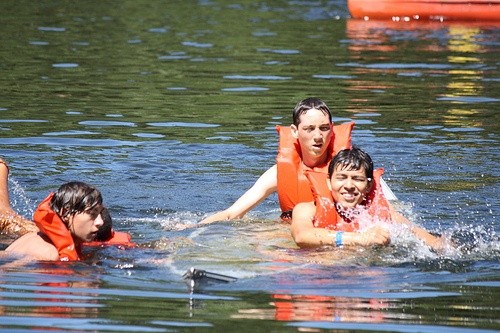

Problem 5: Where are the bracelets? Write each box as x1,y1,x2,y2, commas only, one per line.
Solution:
336,231,344,248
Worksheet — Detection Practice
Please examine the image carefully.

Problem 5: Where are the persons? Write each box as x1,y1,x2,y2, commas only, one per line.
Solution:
290,148,452,254
0,158,132,246
1,182,105,269
197,97,399,225
